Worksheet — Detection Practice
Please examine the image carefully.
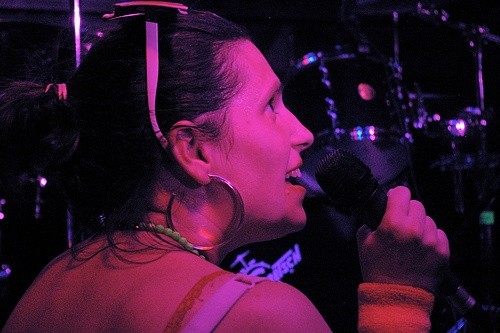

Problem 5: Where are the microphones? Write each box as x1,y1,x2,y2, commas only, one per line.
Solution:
315,149,489,322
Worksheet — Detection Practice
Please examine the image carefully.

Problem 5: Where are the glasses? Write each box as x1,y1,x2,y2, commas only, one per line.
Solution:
103,1,189,153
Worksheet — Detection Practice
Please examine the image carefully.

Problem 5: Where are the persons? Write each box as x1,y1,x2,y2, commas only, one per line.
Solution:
0,0,451,333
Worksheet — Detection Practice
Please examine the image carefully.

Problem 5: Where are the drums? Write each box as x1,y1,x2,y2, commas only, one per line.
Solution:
296,124,410,240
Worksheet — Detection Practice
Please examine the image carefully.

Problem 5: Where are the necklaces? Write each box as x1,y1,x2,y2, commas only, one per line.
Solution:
115,223,207,260
